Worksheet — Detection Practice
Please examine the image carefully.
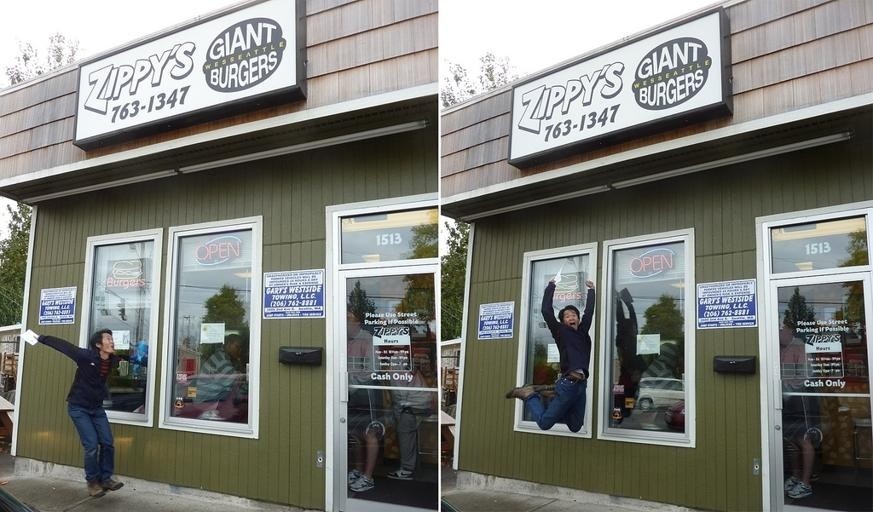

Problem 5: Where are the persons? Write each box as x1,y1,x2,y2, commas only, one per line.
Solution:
780,319,828,499
506,276,596,433
614,288,647,422
347,305,432,492
192,333,247,403
631,343,683,430
33,327,124,499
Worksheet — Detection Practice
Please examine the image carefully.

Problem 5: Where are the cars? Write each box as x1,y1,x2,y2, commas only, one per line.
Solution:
664,400,684,431
130,371,247,424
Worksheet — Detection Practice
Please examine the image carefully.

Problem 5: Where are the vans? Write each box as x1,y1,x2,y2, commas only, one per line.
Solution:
633,376,685,414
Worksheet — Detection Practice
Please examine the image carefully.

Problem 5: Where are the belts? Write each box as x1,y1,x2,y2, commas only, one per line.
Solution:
564,373,587,383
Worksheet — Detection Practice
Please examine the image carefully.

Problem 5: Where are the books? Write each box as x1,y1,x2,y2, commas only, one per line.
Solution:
20,329,39,346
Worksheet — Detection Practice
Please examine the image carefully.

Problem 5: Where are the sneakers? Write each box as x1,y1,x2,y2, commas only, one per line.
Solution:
347,469,375,492
87,477,124,498
810,473,820,481
505,383,534,399
384,469,415,480
534,385,557,397
784,476,812,499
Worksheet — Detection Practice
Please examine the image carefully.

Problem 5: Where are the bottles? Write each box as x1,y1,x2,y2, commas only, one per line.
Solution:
174,392,185,409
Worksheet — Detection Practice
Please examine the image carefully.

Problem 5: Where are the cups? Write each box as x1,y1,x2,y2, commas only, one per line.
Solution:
119,361,129,377
186,359,194,371
